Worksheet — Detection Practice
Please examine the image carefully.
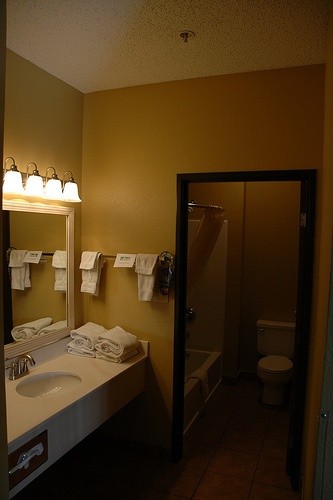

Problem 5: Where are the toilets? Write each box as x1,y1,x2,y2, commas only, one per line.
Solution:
256,319,297,405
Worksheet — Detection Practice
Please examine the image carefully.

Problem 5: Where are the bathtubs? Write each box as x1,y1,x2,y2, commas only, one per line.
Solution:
184,349,223,432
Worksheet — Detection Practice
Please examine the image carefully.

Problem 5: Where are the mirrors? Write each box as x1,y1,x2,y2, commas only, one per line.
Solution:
0,202,75,359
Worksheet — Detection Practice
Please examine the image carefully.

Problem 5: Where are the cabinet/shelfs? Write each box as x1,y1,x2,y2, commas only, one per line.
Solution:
0,430,49,492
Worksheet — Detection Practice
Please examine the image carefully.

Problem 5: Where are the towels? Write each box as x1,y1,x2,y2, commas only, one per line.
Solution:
95,325,140,364
134,253,159,302
185,368,211,400
10,317,53,342
8,249,32,291
36,320,67,337
78,251,105,297
67,321,108,359
51,249,67,291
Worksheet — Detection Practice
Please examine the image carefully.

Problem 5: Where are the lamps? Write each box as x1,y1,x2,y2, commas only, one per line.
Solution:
3,158,82,203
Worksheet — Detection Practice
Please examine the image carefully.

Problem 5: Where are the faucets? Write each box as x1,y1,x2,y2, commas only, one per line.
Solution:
18,353,36,375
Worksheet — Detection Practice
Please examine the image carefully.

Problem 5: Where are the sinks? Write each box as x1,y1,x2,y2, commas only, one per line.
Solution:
15,370,77,399
